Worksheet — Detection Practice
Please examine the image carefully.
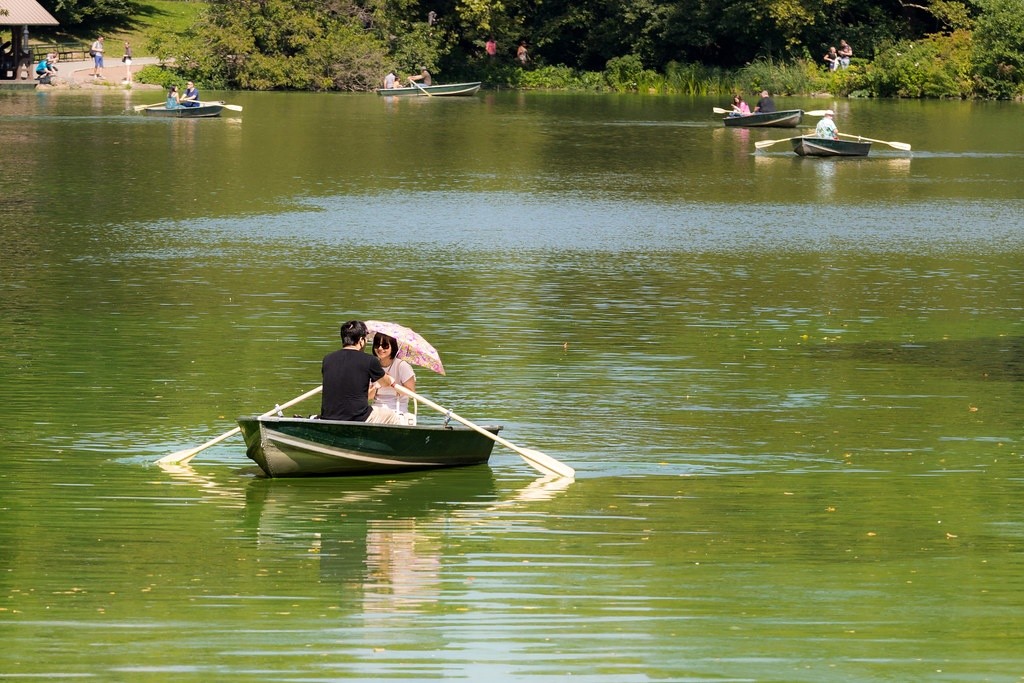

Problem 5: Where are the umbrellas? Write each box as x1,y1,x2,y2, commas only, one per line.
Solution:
363,320,447,377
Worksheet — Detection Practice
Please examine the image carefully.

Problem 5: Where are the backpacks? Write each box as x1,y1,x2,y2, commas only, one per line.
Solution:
89,41,98,57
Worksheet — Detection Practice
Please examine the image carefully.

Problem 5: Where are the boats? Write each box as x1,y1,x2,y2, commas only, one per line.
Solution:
235,414,504,478
145,100,225,117
791,135,873,157
723,109,804,127
377,81,482,97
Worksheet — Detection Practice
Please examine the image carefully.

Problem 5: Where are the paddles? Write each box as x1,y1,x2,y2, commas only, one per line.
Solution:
837,132,912,152
134,102,167,112
803,109,833,117
410,79,432,97
754,133,815,149
712,106,753,115
154,385,323,464
395,383,577,480
188,99,243,112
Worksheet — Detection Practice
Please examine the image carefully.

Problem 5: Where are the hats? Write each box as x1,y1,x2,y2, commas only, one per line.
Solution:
762,91,769,98
825,110,834,115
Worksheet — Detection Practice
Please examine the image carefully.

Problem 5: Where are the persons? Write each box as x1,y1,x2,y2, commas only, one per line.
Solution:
729,94,750,117
367,331,417,425
838,40,853,70
409,65,432,87
166,84,186,109
384,69,397,89
393,77,404,88
123,41,132,84
754,89,777,113
179,80,200,107
517,40,531,64
824,46,838,72
36,50,60,80
816,110,839,140
91,34,105,79
485,32,497,55
320,321,399,425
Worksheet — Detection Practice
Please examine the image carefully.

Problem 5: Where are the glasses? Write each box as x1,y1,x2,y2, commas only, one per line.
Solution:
373,342,391,349
